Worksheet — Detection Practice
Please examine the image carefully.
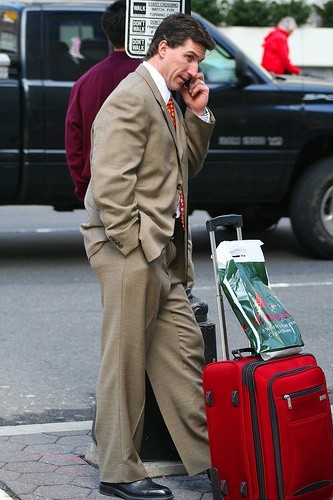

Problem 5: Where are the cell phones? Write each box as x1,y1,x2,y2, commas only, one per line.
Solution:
184,79,192,89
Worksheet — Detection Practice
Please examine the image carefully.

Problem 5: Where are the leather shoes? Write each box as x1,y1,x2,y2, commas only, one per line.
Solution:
100,474,174,500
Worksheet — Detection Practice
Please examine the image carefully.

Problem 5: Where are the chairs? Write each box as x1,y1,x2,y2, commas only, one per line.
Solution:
52,42,75,69
79,39,108,67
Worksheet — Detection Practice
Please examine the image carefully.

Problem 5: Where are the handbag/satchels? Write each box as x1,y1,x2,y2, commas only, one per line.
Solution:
210,238,304,361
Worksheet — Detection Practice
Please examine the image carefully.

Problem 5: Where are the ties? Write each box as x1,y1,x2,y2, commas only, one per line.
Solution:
167,97,186,227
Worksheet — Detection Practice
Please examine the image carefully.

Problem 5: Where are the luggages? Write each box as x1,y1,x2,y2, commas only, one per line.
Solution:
205,214,333,500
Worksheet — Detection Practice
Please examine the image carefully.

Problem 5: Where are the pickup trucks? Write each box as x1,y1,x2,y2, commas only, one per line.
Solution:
0,0,333,261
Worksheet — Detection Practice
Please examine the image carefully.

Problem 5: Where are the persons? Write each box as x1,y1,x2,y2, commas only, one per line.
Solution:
261,15,302,77
79,12,217,500
66,0,146,202
